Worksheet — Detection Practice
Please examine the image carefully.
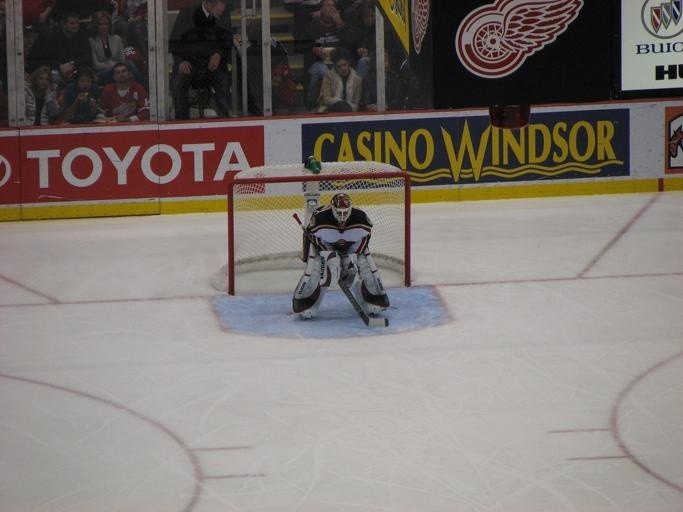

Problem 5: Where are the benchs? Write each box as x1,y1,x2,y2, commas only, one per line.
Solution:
0,1,434,127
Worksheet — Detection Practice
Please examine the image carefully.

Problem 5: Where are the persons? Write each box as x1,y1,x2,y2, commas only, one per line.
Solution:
0,2,430,128
291,194,391,320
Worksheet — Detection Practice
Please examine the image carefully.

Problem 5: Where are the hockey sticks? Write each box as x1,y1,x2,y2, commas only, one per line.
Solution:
292,213,389,327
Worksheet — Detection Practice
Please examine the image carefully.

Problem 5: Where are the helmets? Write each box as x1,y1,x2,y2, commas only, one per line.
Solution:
331,192,352,226
